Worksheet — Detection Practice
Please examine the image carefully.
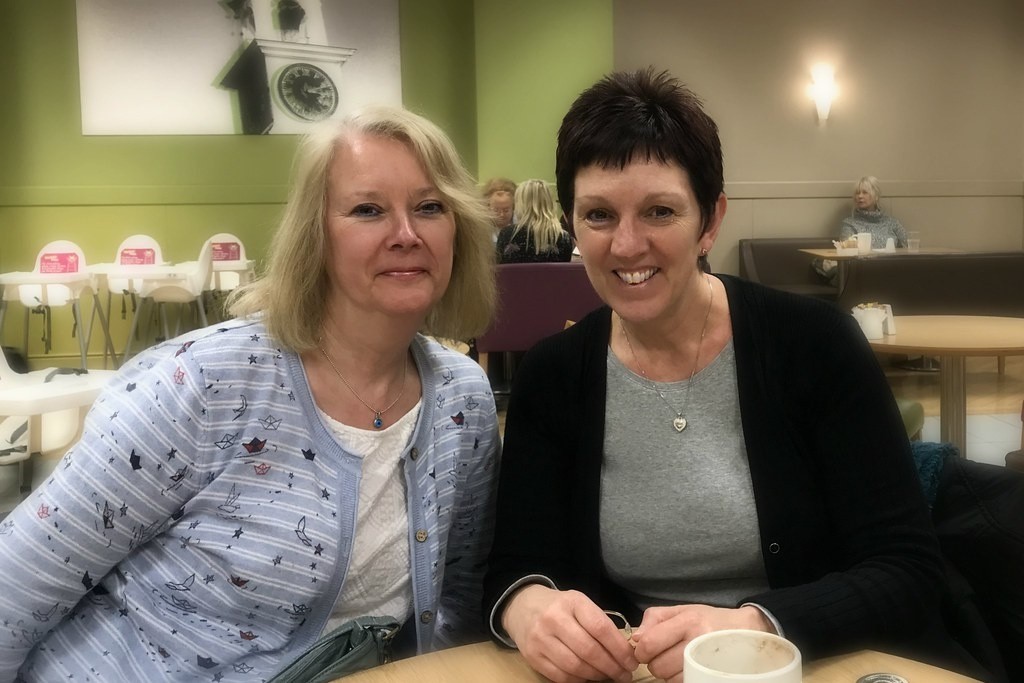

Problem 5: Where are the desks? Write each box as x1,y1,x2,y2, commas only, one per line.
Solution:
0,259,254,499
862,314,1024,454
797,248,966,371
322,634,980,683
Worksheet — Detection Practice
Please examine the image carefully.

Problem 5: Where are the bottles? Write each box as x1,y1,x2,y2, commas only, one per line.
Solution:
885,238,896,254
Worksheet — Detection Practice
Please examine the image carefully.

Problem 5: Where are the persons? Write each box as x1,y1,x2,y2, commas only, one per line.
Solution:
482,64,942,683
840,176,908,249
495,178,573,264
0,107,504,683
480,176,516,244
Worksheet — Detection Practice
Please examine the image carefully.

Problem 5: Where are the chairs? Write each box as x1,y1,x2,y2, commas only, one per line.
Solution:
18,234,246,371
470,259,608,383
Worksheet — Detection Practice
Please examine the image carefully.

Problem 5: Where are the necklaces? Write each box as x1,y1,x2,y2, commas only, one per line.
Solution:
619,275,713,432
322,348,407,429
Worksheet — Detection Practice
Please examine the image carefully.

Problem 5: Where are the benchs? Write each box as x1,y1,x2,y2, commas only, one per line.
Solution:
738,236,842,297
837,252,1024,371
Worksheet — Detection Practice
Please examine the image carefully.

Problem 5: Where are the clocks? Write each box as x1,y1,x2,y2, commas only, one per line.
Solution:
270,62,339,124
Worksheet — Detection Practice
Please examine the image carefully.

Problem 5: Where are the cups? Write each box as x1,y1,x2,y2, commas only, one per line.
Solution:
683,628,802,683
907,232,920,251
852,233,871,254
